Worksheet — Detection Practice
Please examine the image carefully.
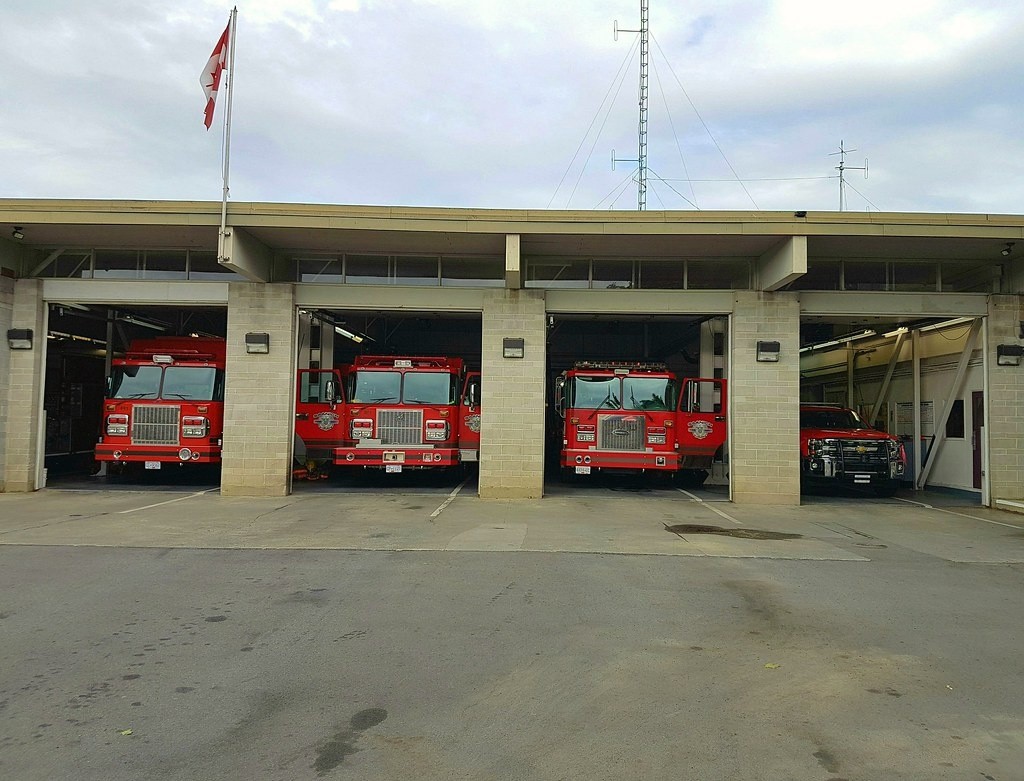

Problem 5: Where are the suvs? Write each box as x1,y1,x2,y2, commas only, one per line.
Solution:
800,401,906,497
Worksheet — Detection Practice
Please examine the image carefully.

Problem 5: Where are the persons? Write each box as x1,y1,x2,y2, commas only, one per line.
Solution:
305,460,332,483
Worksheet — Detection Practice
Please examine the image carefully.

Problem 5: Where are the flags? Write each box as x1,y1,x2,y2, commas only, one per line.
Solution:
199,18,230,132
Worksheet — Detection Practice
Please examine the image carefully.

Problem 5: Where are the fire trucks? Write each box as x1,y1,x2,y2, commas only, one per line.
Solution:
295,355,480,489
93,335,227,484
554,361,727,488
